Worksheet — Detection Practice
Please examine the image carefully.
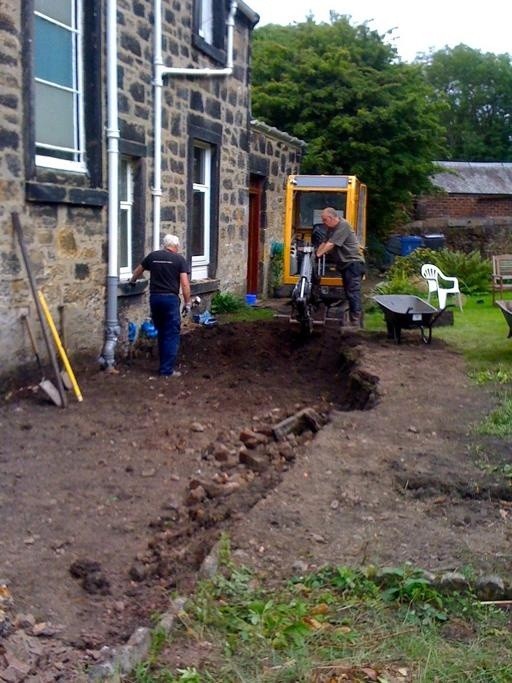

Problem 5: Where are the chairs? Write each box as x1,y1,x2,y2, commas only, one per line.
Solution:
492,252,512,305
420,264,464,313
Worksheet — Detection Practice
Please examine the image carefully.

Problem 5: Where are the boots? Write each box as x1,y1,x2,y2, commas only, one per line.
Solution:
350,311,364,328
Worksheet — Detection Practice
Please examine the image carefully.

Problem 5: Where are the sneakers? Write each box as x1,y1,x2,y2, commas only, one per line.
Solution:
164,371,181,379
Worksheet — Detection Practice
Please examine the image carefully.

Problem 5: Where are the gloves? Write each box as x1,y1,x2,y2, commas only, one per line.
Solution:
182,304,190,317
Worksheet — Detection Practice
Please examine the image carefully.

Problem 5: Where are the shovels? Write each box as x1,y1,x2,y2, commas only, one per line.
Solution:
25,316,63,408
57,306,75,390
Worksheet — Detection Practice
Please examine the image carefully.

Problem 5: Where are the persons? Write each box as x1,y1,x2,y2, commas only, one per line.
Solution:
311,206,367,327
125,233,191,379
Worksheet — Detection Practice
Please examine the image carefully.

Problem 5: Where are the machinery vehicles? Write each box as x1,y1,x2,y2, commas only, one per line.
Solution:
284,171,367,336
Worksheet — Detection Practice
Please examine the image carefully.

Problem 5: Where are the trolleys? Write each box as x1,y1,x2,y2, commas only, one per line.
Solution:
375,293,454,344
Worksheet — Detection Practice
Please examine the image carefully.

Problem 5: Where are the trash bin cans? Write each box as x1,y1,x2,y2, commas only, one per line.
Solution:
400,234,444,256
